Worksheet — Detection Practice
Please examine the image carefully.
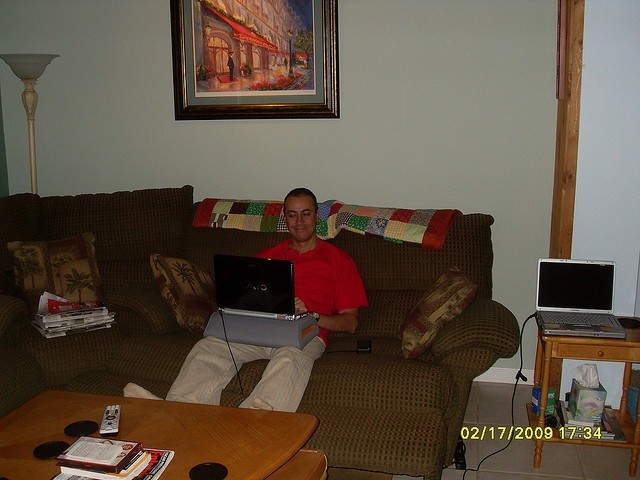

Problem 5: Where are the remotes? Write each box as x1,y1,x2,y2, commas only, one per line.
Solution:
100,404,122,438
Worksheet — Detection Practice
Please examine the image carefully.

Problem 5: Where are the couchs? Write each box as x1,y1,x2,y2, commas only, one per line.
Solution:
0,185,520,479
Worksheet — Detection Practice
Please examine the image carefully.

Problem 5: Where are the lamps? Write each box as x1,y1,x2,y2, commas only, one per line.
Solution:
1,53,61,193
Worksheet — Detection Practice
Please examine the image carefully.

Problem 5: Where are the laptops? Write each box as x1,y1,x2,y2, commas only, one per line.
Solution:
213,254,313,321
536,257,625,339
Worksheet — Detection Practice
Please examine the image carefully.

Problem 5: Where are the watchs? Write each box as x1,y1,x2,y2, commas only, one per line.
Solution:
312,311,320,322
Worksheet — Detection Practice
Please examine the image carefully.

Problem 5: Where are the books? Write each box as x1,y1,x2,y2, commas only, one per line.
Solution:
556,401,628,444
50,435,175,480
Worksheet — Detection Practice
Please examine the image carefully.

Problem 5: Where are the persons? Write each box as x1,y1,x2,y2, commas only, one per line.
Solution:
166,187,367,413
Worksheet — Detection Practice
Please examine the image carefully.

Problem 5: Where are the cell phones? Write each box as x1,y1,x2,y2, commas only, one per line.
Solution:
356,338,371,353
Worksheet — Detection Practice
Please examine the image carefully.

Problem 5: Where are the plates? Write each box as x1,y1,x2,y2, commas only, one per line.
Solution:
64,420,99,437
189,462,228,479
33,441,70,460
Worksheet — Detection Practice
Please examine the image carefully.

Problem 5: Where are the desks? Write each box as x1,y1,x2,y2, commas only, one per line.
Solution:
527,316,640,477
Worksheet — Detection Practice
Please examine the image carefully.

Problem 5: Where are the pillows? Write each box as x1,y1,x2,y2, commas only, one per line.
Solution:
7,231,103,312
396,265,477,360
149,252,219,332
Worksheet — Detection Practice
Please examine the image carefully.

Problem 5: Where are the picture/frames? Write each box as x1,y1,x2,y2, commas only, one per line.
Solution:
170,0,340,120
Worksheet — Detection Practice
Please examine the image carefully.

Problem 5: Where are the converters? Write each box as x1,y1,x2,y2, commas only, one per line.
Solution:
454,434,466,469
